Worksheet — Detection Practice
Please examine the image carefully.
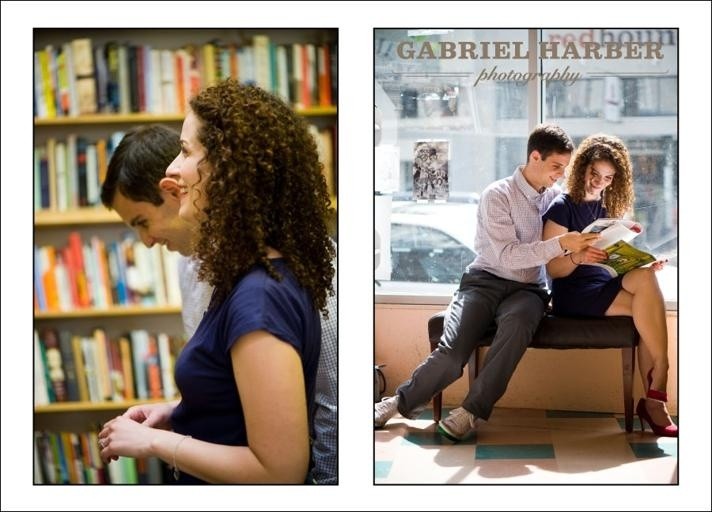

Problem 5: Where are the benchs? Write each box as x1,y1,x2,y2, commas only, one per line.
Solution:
428,309,640,433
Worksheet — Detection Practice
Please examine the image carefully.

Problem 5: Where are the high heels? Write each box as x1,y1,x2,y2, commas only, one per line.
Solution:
636,367,678,436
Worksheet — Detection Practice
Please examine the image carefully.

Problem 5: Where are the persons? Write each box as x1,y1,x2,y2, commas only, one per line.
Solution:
375,124,601,441
542,132,678,437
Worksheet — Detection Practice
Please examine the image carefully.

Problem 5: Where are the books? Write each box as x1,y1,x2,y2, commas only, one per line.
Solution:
566,216,644,250
580,238,678,278
32,33,337,118
33,133,180,485
305,125,336,196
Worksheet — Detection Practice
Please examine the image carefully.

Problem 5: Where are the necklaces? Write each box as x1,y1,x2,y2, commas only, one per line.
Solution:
585,197,598,222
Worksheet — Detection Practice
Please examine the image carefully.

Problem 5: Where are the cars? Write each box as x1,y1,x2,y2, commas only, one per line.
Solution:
374,200,479,285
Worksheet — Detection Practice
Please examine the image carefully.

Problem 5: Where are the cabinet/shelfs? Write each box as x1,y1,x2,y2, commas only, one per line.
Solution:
33,106,338,414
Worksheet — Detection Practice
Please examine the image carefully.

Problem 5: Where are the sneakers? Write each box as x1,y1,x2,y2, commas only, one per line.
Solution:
374,396,400,427
437,407,479,442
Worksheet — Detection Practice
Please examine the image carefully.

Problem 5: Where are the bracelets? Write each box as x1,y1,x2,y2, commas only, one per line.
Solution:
568,253,581,268
